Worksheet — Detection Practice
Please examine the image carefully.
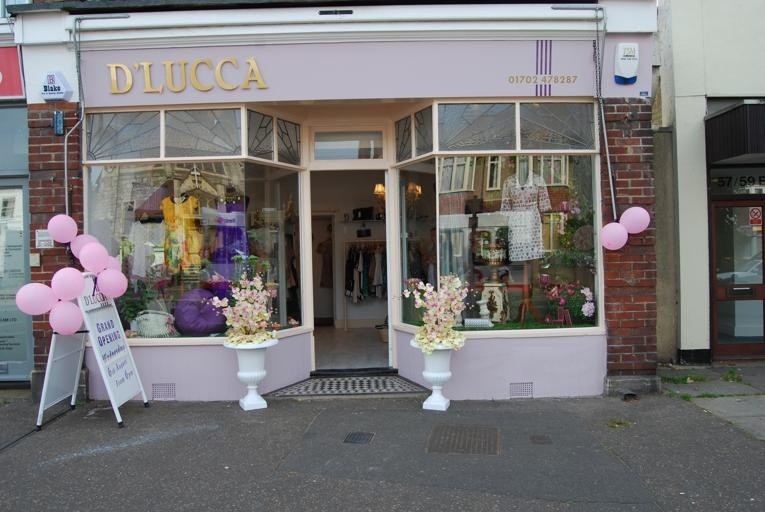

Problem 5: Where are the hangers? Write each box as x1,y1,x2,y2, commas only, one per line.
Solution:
351,243,387,254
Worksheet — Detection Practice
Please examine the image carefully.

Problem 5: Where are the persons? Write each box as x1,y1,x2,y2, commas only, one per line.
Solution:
158,196,205,274
416,228,438,291
287,255,299,304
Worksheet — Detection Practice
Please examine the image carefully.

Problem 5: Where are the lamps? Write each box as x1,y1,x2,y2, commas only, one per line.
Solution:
373,179,423,203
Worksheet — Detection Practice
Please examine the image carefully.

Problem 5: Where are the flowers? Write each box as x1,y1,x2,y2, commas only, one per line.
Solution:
118,291,149,321
546,281,595,320
402,273,470,355
203,268,277,344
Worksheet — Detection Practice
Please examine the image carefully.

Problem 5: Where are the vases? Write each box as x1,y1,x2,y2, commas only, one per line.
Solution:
409,336,453,411
128,318,138,331
222,339,279,411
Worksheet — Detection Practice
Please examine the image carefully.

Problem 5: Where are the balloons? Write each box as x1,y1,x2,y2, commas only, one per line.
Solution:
14,282,59,316
49,301,83,335
620,206,651,235
69,232,129,298
600,223,628,250
51,268,86,300
47,213,77,243
